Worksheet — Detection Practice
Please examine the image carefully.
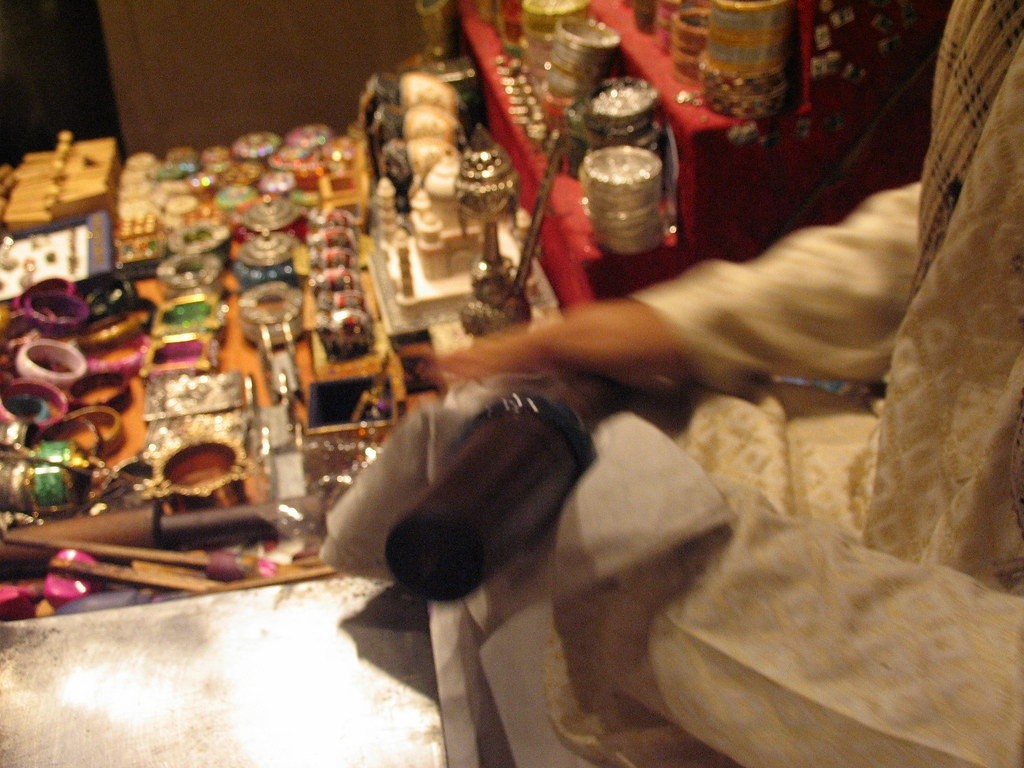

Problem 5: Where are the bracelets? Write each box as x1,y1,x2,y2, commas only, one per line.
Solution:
0,268,155,458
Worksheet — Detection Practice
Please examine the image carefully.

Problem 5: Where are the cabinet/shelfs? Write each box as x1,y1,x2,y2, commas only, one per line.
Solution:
405,3,816,311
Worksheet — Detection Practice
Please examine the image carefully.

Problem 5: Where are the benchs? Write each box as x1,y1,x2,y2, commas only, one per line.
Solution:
0,556,455,768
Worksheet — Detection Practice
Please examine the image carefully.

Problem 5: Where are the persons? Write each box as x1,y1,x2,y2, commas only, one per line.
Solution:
437,0,1024,768
